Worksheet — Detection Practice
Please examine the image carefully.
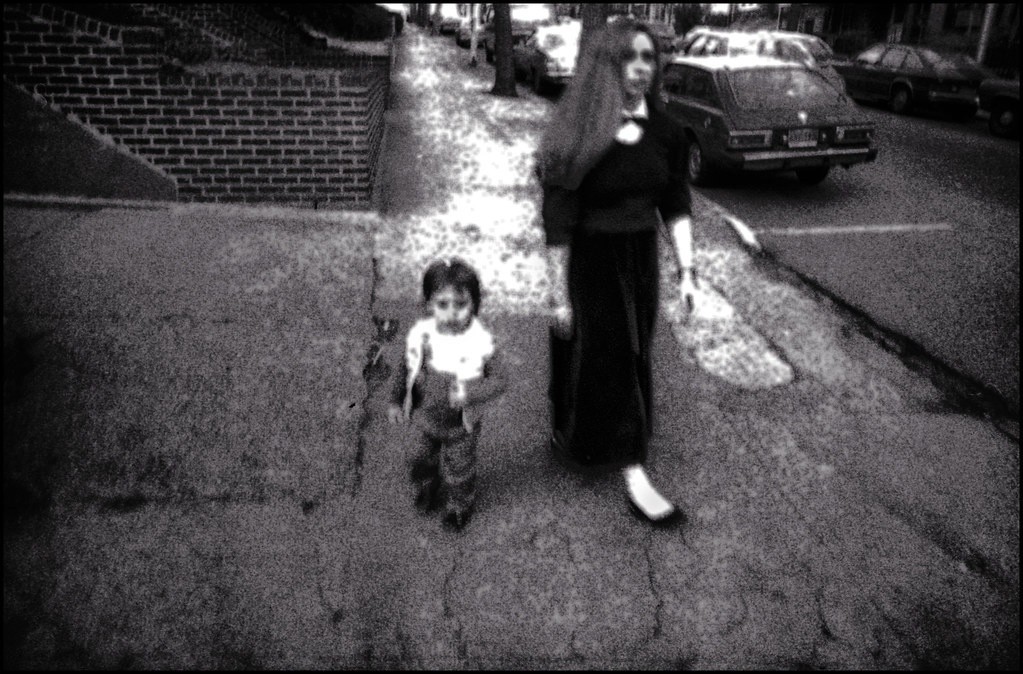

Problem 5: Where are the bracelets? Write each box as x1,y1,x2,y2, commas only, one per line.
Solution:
677,266,698,282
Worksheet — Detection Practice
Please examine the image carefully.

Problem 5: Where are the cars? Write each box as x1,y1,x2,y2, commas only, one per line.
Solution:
420,3,581,98
645,56,879,193
677,24,845,91
830,43,984,122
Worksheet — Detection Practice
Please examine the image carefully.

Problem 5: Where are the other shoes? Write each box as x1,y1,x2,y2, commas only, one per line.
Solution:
443,505,474,530
415,474,446,509
626,490,683,528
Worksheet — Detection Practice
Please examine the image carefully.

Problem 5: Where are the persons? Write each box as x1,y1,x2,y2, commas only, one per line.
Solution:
529,19,702,526
386,257,510,530
